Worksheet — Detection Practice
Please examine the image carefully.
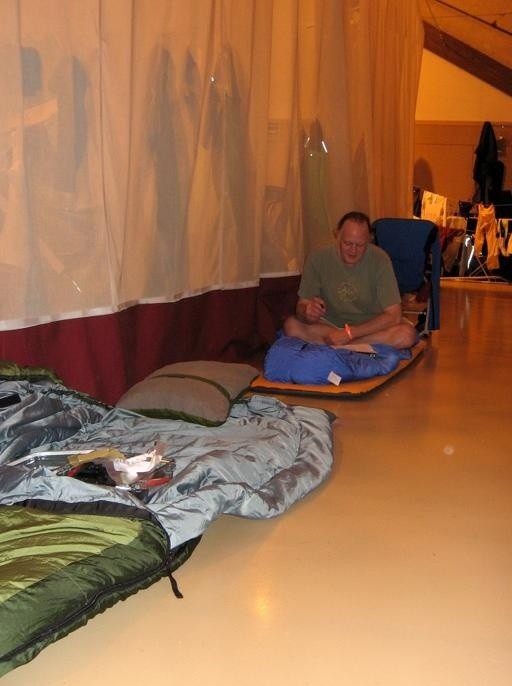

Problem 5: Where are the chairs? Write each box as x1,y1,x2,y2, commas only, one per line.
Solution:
371,217,438,337
464,236,491,282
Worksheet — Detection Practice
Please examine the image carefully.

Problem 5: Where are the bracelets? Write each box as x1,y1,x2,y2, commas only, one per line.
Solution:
344,323,353,341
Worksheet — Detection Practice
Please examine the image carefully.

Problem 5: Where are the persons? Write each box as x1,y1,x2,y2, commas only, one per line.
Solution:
281,211,417,355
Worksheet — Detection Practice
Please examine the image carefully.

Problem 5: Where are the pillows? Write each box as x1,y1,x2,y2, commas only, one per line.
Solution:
114,361,260,427
